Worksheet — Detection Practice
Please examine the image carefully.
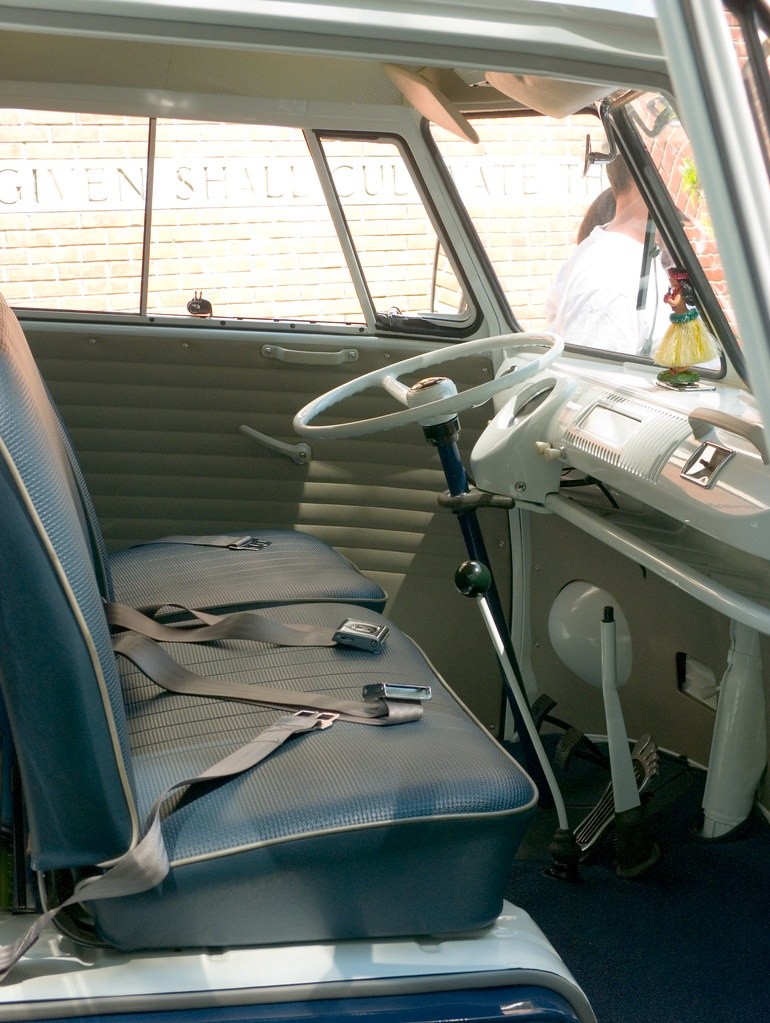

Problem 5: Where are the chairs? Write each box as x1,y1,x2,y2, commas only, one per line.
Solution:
0,291,540,953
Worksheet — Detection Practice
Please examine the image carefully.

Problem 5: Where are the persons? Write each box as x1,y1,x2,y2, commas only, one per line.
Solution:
542,134,721,371
